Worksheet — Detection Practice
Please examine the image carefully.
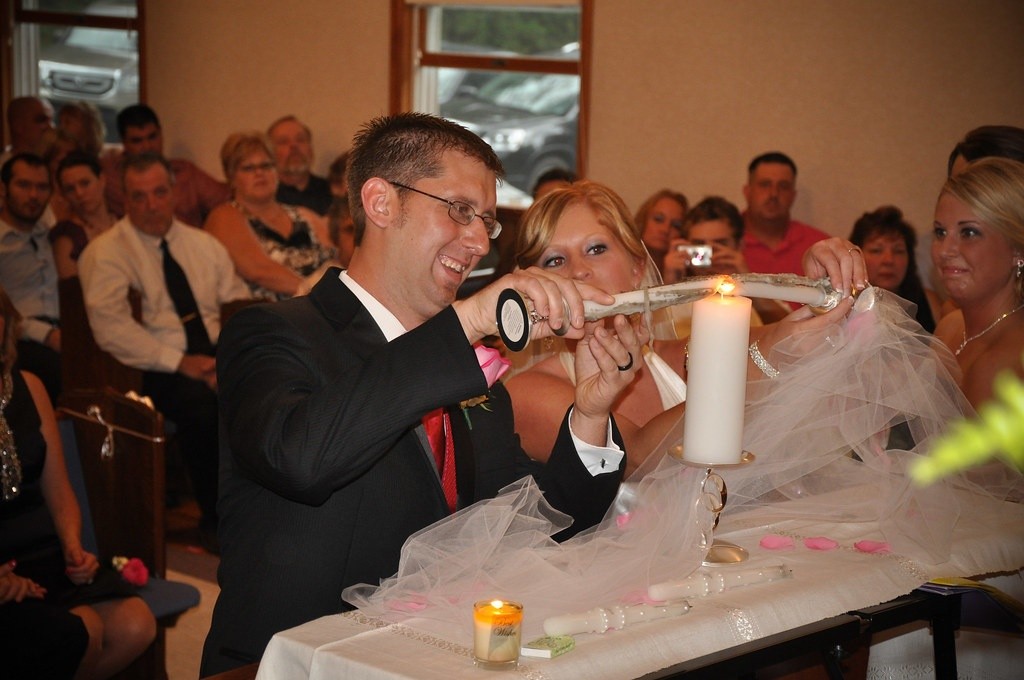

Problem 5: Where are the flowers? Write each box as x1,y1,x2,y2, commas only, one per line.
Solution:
456,342,513,430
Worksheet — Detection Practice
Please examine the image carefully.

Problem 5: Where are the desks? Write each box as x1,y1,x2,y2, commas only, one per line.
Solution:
254,494,1024,680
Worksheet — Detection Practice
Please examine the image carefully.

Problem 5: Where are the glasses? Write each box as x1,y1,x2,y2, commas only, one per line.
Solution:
389,181,503,239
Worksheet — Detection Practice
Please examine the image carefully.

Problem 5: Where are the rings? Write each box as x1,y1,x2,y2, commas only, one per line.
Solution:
824,335,836,349
617,351,633,371
848,247,859,256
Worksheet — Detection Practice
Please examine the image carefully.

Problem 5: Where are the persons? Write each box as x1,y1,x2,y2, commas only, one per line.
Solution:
638,150,836,324
913,125,1024,473
531,165,578,204
505,180,867,480
198,113,655,679
850,205,942,341
0,295,157,680
0,95,357,552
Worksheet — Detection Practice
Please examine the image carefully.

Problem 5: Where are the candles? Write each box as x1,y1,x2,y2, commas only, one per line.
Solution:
575,272,828,462
542,566,795,636
472,599,524,670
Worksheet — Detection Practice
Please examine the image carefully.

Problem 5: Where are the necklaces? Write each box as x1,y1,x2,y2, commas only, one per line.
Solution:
955,304,1024,356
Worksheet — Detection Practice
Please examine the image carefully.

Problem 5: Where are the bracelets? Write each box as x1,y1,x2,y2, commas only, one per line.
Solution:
749,342,780,378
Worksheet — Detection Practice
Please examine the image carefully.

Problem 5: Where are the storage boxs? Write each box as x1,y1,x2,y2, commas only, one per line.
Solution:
521,636,574,658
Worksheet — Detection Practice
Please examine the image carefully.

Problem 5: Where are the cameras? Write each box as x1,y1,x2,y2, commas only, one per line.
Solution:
677,244,712,268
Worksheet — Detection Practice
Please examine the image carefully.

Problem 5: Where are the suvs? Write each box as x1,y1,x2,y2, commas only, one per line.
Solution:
38,2,139,137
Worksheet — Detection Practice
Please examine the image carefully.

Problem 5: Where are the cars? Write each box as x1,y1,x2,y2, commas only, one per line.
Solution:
437,38,581,200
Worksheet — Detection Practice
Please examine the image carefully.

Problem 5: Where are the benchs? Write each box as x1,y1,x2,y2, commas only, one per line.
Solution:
49,281,217,680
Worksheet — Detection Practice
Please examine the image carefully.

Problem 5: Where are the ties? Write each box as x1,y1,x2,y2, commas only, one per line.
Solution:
160,239,215,358
424,407,446,480
